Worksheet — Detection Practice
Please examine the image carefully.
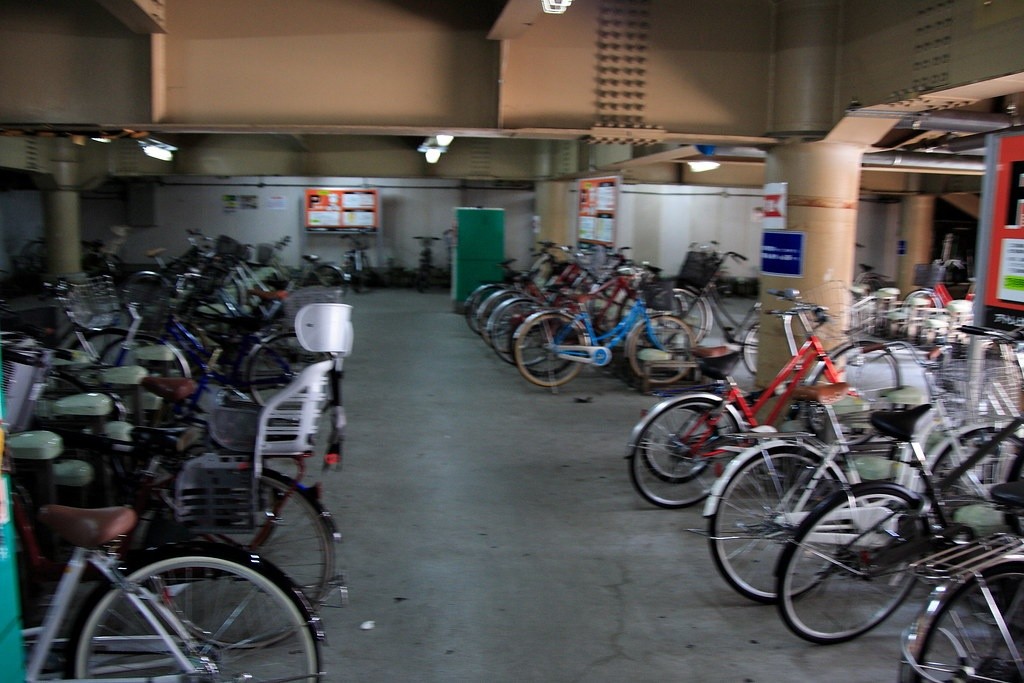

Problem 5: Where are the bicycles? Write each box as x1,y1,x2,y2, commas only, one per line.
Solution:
461,241,1024,683
413,236,441,292
0,228,379,683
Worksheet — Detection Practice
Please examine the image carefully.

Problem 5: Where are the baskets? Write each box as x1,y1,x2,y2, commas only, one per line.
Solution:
285,286,342,327
1,345,54,432
925,356,1023,420
69,275,120,329
948,449,1018,489
911,262,939,289
643,281,673,314
680,251,721,286
257,242,274,262
